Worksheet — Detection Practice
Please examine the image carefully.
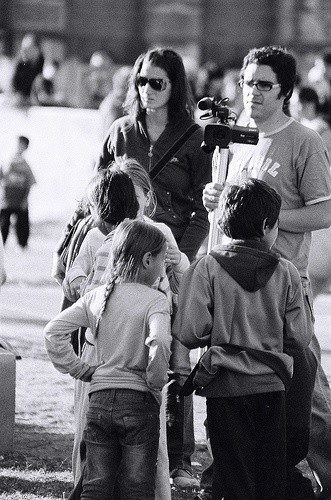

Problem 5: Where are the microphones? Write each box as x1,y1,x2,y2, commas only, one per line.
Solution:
196,96,217,113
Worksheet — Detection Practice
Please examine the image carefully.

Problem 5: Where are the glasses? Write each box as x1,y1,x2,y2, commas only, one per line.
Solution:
238,79,282,91
136,73,170,92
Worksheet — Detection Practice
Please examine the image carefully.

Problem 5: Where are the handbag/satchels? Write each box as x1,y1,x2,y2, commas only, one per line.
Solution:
52,204,96,287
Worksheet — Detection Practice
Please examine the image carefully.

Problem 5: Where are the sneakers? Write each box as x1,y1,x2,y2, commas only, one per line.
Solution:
169,468,201,494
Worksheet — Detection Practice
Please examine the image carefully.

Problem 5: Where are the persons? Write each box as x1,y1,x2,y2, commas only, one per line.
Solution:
94,46,213,496
0,136,36,252
169,177,315,500
43,155,190,500
202,44,331,500
0,31,331,154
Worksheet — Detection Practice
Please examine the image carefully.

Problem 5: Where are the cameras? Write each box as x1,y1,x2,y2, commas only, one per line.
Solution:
202,122,259,149
